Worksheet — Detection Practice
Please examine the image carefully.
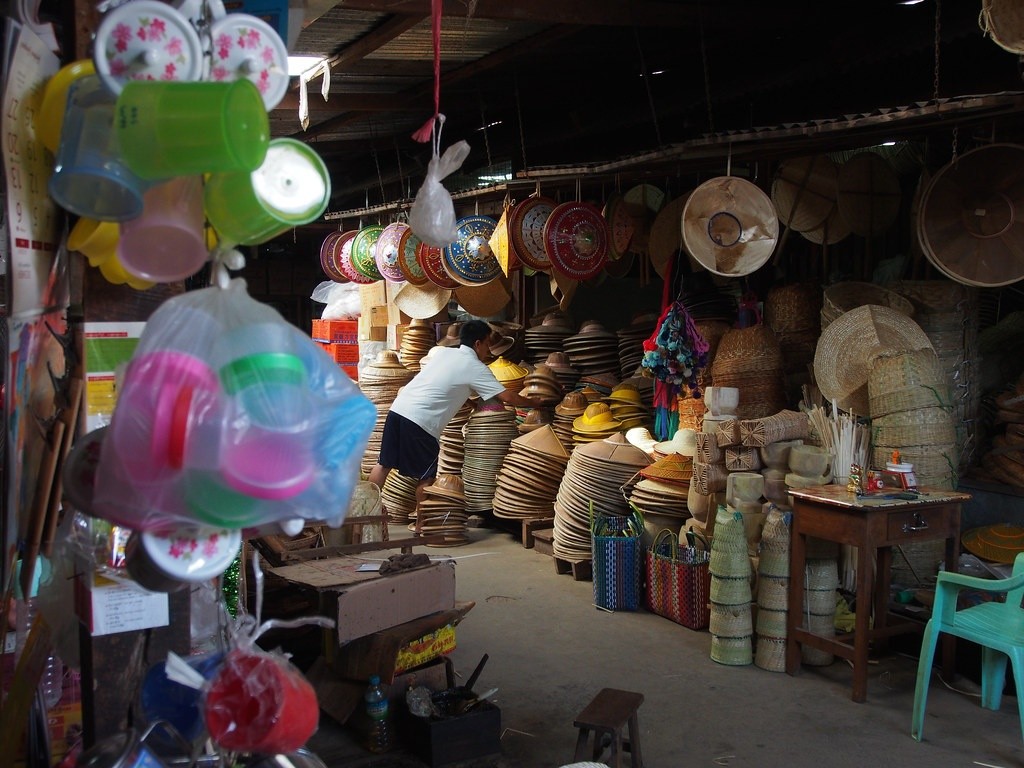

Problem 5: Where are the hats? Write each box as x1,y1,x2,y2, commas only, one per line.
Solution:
915,143,1024,288
961,522,1024,563
681,175,780,279
677,269,737,320
319,189,700,289
359,309,694,561
812,303,939,420
981,376,1024,489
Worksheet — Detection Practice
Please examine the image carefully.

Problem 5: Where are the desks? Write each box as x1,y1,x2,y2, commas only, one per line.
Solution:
783,484,973,703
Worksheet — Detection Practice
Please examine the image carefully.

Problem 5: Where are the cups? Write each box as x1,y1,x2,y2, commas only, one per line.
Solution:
36,0,331,281
205,658,318,753
142,652,227,747
92,348,376,592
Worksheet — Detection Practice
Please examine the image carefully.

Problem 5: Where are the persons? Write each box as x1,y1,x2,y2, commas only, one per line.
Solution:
368,320,545,537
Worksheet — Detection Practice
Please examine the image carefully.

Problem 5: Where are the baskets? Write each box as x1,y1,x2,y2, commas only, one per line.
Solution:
708,503,840,666
676,280,915,421
646,527,710,631
590,499,647,613
868,347,983,587
693,399,871,496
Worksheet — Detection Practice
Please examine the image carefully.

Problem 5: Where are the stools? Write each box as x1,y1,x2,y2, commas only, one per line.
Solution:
571,688,645,768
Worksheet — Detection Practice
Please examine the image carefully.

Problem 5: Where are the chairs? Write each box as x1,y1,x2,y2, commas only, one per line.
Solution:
910,551,1024,744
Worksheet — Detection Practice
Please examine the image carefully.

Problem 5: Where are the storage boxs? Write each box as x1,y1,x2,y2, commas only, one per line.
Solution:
265,546,458,662
321,662,449,735
396,696,502,768
73,320,174,637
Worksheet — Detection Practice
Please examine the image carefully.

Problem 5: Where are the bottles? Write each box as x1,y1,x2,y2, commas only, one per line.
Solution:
364,674,391,752
